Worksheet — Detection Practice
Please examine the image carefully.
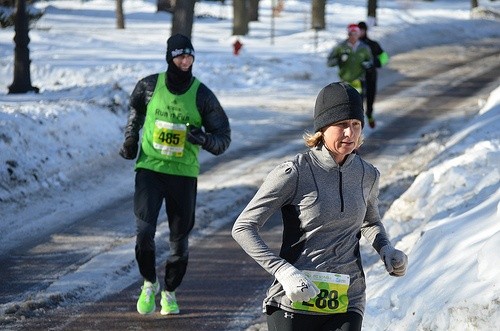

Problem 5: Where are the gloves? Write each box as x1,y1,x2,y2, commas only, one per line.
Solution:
119,142,138,160
187,125,206,144
381,245,408,277
275,262,320,302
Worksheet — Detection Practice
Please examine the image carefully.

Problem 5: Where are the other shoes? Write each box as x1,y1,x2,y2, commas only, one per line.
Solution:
368,116,375,129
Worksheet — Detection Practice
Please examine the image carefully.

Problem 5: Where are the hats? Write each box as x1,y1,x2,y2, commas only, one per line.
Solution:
348,25,361,35
314,82,364,133
166,33,194,63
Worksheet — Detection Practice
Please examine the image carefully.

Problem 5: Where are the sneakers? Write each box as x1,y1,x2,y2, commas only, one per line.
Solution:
137,276,160,314
160,291,179,315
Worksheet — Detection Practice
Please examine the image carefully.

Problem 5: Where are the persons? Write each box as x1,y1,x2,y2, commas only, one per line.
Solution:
327,22,388,128
119,34,231,315
232,82,408,331
